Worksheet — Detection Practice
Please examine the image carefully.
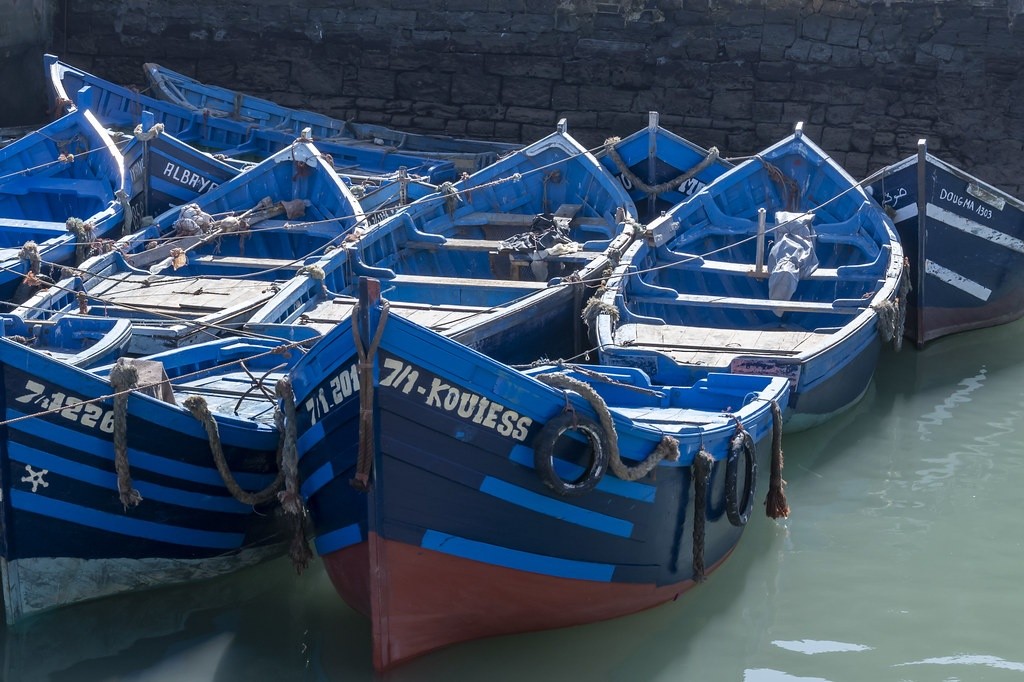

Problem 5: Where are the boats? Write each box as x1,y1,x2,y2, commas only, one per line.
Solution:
0,47,741,566
591,118,908,438
859,138,1023,351
352,271,792,676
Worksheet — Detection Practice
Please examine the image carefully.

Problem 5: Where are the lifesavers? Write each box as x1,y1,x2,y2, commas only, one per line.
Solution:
534,409,611,498
724,429,759,527
